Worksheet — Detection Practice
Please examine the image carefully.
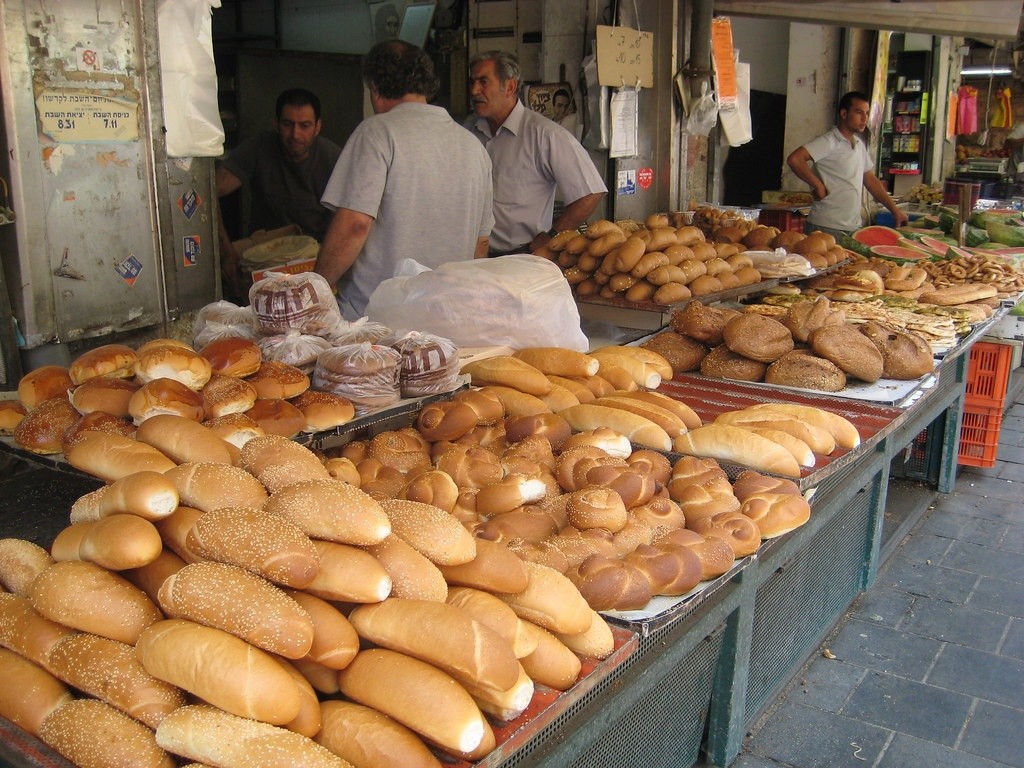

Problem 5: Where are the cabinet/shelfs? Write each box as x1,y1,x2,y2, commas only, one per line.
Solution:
889,50,930,174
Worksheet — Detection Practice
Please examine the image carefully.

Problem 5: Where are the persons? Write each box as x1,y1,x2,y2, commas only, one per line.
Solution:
786,90,909,246
318,41,496,324
550,88,576,121
216,86,347,277
457,50,607,261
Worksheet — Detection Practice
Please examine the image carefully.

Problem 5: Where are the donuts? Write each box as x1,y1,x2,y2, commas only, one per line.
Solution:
916,257,1024,301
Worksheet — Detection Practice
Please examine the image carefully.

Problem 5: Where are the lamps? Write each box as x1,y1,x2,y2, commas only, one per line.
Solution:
960,47,1014,75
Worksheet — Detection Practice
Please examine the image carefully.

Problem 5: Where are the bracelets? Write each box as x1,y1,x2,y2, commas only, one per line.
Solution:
547,226,560,238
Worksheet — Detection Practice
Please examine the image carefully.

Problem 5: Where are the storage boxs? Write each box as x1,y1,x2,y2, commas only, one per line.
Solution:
897,314,1024,468
759,209,806,234
230,226,338,309
577,298,750,331
877,178,995,228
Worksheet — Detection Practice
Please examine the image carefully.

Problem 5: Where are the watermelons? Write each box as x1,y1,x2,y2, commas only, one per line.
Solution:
838,209,1024,265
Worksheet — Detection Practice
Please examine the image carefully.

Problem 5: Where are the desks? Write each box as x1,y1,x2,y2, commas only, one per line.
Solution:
0,346,970,768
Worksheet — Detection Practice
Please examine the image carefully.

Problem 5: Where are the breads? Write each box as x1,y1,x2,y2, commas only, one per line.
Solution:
532,205,847,305
638,298,934,391
742,254,1001,347
0,272,862,768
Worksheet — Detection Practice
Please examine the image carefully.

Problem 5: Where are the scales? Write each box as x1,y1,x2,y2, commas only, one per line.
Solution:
963,156,1009,174
